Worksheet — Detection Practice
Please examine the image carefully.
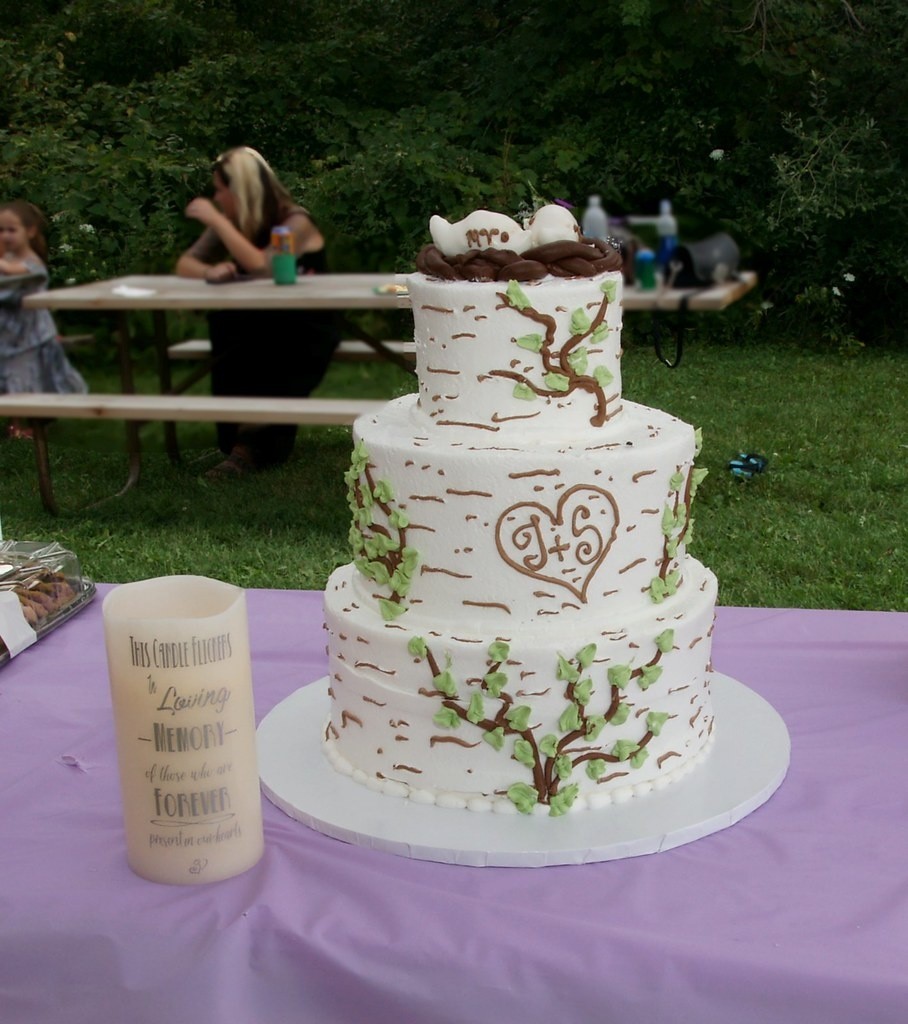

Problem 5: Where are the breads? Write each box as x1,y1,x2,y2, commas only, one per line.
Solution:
0,567,74,649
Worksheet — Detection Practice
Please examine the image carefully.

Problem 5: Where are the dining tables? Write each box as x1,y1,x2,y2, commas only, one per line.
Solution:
1,577,907,1024
24,269,756,475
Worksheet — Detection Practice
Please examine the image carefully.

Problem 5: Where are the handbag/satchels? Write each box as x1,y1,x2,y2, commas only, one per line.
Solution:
673,233,739,287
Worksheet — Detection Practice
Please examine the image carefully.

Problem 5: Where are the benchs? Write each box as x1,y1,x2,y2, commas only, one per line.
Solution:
0,390,391,521
168,337,422,392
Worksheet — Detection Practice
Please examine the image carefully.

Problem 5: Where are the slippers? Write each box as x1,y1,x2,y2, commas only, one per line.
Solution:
728,453,770,483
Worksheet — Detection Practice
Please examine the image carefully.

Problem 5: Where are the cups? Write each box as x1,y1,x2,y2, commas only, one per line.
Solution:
635,248,657,289
102,574,264,890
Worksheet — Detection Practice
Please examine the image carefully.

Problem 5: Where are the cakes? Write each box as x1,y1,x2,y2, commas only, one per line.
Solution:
321,204,719,817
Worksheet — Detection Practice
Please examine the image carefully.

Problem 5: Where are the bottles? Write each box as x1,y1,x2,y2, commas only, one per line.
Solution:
654,200,679,265
583,196,609,245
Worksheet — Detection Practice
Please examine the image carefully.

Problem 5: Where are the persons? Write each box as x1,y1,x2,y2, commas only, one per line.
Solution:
0,196,90,394
174,145,343,481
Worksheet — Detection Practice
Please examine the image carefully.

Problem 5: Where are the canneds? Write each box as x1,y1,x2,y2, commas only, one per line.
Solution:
270,225,295,284
